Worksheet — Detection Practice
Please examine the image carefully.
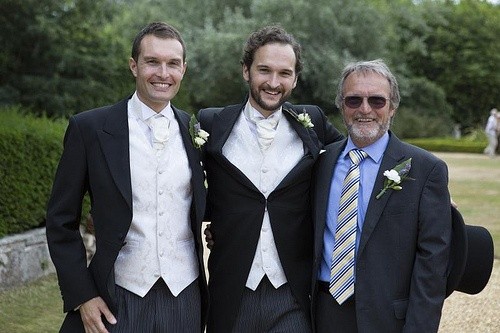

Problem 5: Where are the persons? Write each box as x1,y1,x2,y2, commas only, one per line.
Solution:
203,59,452,333
495,111,500,156
47,21,207,333
194,25,459,333
483,108,500,157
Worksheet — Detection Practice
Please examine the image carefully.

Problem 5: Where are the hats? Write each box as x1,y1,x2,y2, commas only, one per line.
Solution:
445,203,495,299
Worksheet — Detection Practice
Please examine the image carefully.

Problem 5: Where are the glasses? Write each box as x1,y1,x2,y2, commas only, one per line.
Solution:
339,95,391,111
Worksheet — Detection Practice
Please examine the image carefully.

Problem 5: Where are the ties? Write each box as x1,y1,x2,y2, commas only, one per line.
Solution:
249,118,277,155
147,116,171,151
329,149,368,305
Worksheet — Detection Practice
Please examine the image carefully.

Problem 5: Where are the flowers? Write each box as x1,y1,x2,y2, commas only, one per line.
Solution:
376,158,416,200
297,113,314,128
188,114,209,149
